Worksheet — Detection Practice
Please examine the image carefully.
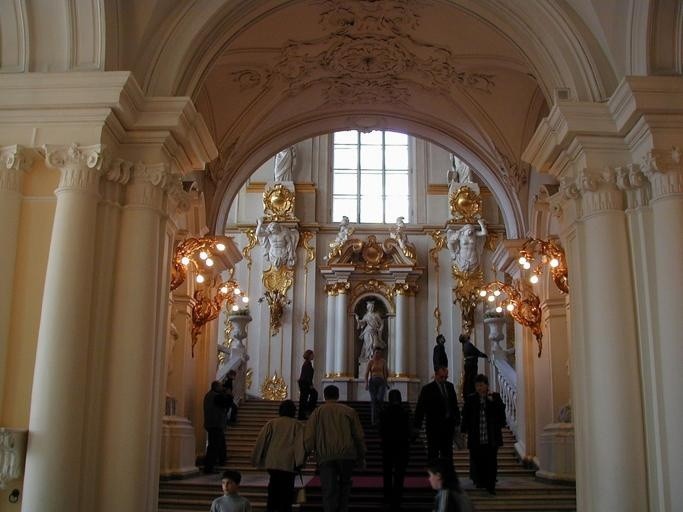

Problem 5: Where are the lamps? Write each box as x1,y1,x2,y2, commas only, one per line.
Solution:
191,280,249,357
480,282,543,358
518,237,571,294
167,236,226,290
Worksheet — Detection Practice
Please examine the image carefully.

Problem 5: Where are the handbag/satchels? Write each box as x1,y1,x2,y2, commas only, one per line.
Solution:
295,467,306,504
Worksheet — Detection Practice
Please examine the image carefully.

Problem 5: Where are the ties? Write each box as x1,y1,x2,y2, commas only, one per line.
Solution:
440,383,448,401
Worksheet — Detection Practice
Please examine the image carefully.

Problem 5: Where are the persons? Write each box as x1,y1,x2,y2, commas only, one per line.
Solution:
447,218,488,274
204,334,506,511
255,217,294,270
355,302,384,359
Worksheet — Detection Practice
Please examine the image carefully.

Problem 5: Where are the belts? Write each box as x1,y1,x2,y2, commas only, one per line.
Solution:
466,356,474,358
